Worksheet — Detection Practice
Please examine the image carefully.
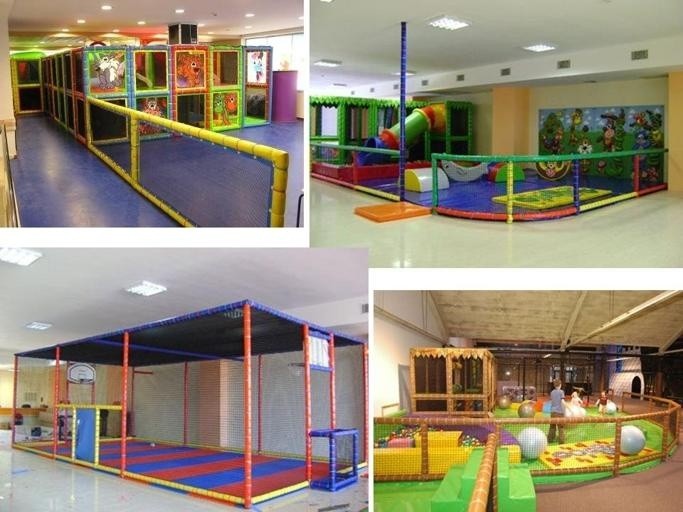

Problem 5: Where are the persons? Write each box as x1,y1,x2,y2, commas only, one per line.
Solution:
547,377,565,444
570,389,583,406
397,152,526,194
374,424,485,448
593,390,607,416
